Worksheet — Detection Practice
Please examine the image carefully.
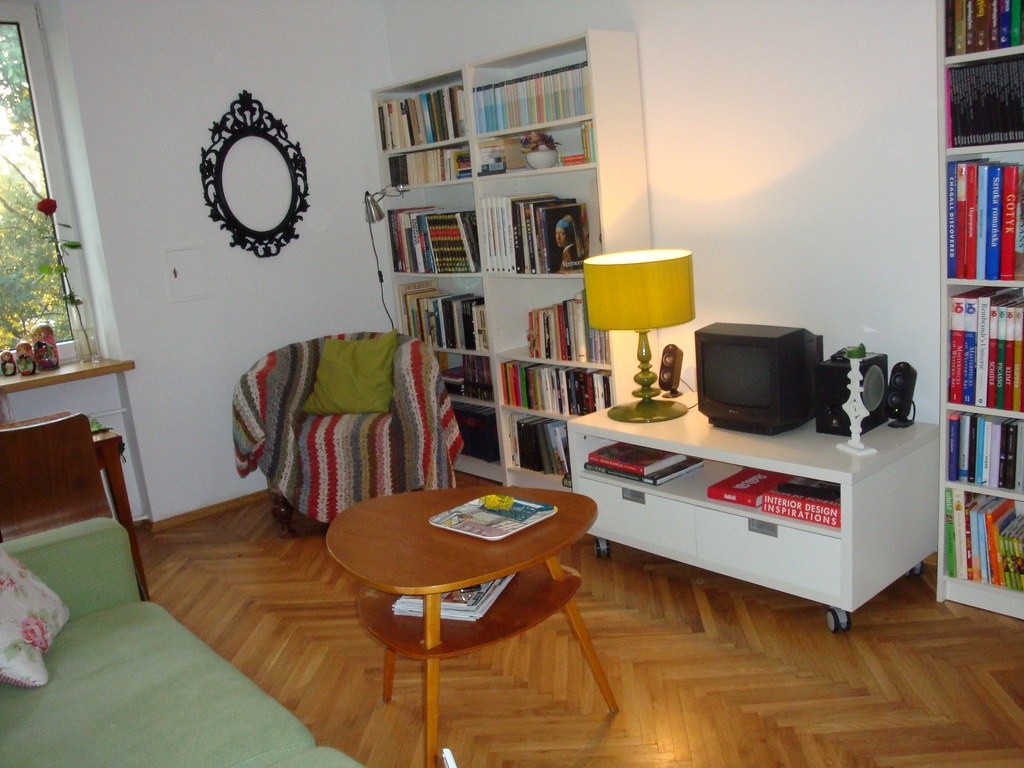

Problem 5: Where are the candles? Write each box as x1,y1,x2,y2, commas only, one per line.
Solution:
846,342,867,358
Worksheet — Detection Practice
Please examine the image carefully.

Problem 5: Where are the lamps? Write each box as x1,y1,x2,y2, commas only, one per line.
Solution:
362,190,388,223
583,248,696,424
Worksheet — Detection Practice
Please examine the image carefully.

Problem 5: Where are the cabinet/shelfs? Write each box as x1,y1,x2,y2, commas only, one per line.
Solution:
370,27,663,492
935,0,1024,621
566,391,939,635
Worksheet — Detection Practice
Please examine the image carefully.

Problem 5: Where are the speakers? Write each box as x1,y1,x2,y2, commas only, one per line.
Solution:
657,344,684,398
884,361,916,427
815,353,890,437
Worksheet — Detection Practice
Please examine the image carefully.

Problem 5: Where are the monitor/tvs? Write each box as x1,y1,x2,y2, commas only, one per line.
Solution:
693,321,824,436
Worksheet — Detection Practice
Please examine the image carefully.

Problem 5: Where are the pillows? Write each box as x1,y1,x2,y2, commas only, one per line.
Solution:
0,547,70,688
302,329,400,416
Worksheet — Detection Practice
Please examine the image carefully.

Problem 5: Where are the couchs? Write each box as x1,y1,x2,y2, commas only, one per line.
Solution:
0,515,365,768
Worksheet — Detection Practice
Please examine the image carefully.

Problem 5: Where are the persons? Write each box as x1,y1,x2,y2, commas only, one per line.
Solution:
556,215,586,273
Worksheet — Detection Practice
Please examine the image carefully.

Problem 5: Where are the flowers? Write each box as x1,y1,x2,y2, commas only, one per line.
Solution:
37,197,78,305
520,131,562,154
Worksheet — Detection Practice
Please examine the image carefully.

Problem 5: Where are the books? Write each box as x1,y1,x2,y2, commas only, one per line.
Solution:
584,443,704,488
378,61,612,474
944,0,1024,593
707,468,841,528
392,573,516,622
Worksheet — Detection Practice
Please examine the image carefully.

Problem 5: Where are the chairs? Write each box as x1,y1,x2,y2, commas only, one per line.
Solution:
231,331,465,540
0,414,150,602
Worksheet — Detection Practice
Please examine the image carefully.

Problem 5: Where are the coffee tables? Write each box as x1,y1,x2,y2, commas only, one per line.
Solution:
326,485,620,768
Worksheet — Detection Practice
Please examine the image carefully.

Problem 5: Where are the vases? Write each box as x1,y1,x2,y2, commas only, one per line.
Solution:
525,145,559,170
68,295,101,364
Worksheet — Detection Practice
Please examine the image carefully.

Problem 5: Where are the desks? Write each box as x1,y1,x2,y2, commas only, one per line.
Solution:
92,431,149,600
0,357,136,424
